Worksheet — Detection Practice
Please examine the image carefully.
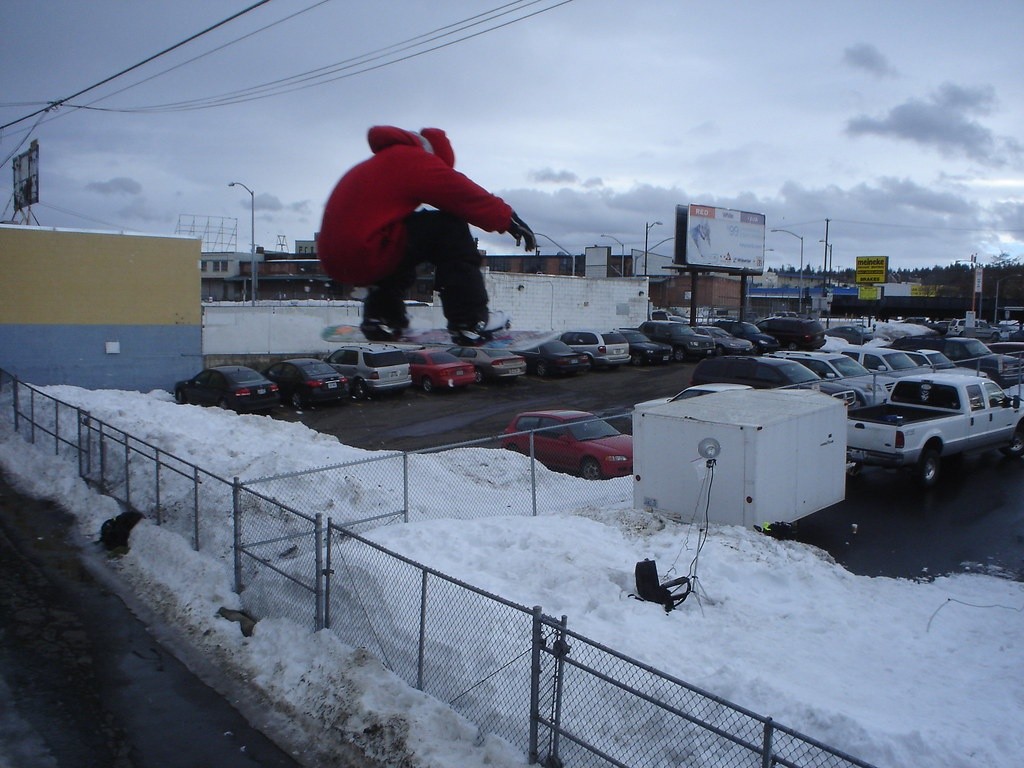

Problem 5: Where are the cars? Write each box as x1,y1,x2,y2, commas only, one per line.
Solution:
650,310,691,321
260,358,349,411
445,346,527,385
403,350,476,392
691,327,753,356
633,383,754,409
500,410,633,480
174,365,281,415
509,340,592,377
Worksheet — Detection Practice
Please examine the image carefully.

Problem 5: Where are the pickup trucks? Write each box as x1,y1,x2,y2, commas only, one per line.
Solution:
847,373,1024,486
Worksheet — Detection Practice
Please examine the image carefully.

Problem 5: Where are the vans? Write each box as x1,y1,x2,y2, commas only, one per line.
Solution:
755,318,827,351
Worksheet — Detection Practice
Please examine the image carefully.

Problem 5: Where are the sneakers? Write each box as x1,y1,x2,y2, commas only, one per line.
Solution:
361,318,402,341
448,321,493,346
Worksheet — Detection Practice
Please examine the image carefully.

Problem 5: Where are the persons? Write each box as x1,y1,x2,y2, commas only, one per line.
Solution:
318,125,536,347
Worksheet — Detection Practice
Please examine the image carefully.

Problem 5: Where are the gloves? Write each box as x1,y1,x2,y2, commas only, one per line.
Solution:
506,208,536,252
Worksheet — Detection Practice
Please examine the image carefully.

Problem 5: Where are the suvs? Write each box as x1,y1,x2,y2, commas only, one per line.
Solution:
692,311,1024,409
609,330,674,366
639,321,715,361
552,328,632,370
323,343,413,399
712,320,781,356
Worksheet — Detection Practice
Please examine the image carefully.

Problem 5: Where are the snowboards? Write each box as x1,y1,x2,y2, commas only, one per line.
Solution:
320,324,567,353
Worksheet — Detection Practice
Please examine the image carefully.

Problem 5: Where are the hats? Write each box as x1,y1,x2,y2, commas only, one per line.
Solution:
420,128,454,168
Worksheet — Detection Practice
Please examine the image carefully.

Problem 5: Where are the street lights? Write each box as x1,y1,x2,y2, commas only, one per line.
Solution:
228,182,255,307
752,249,774,282
771,229,804,313
601,234,624,277
819,240,832,288
994,274,1022,323
645,221,663,275
957,259,984,318
824,219,831,287
